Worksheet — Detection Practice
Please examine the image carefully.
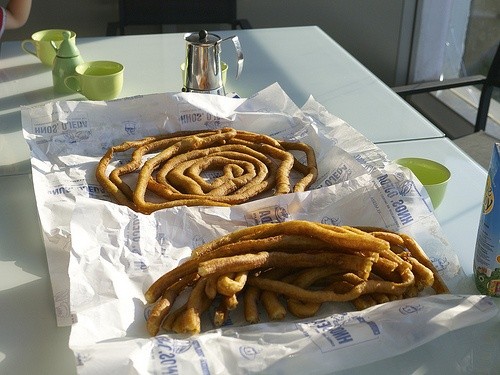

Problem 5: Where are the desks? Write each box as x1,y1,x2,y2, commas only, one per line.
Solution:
0,24,500,375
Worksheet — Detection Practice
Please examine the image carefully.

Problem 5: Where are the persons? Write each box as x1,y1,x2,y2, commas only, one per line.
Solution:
0,0,32,37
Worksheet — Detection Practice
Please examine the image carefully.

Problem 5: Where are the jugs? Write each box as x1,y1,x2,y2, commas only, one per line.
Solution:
184,31,244,96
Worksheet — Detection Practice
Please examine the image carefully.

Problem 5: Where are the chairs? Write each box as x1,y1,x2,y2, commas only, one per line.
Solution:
105,0,253,37
390,42,500,170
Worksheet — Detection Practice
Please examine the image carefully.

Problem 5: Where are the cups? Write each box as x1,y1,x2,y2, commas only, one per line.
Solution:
64,60,125,101
21,29,75,65
393,157,452,210
180,62,228,87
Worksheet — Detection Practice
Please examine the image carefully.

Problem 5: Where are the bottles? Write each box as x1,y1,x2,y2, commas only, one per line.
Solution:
50,32,84,93
